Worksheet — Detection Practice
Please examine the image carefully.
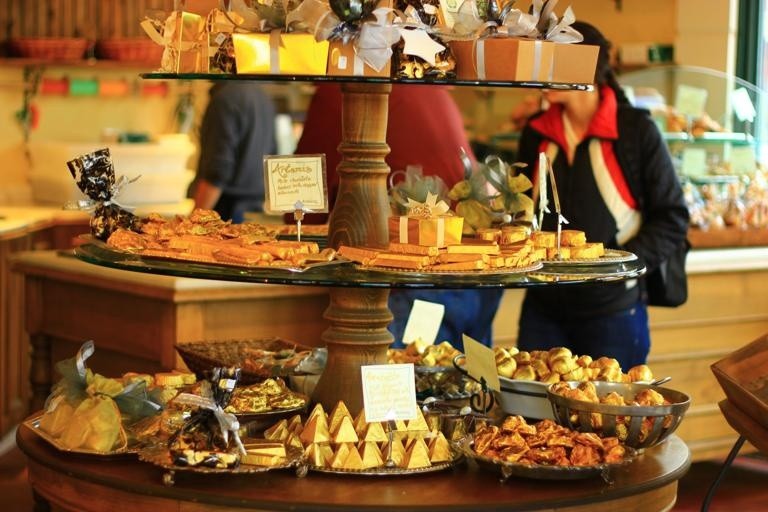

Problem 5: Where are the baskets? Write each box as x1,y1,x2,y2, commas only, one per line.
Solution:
14,39,164,62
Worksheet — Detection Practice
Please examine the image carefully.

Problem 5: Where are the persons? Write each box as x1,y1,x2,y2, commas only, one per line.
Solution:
292,83,481,345
187,80,277,223
513,21,690,375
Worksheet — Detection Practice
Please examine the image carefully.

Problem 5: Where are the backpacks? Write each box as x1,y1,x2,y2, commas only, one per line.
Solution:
521,108,692,309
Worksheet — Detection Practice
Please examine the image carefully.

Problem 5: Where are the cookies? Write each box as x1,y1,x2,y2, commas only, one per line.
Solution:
38,209,675,471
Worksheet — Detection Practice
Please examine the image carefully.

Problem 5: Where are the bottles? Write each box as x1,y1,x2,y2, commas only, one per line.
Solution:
478,374,654,422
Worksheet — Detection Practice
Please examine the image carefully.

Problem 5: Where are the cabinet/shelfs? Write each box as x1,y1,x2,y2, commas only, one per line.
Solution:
0,71,768,511
